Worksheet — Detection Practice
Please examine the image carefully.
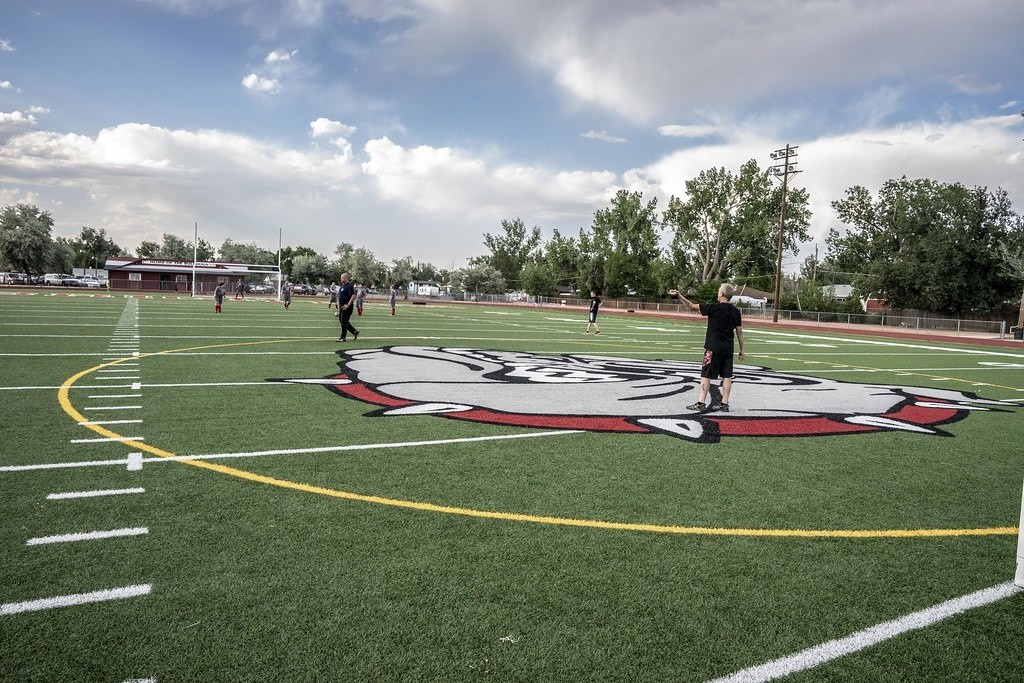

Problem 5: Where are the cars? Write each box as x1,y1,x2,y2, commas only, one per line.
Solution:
291,285,317,295
250,285,275,294
37,273,110,288
0,272,36,285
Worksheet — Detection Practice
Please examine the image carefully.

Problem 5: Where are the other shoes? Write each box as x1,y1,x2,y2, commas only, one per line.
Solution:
585,331,589,334
353,330,359,339
594,331,600,336
336,336,346,342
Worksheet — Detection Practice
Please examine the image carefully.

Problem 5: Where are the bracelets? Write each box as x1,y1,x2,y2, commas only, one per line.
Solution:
347,304,350,306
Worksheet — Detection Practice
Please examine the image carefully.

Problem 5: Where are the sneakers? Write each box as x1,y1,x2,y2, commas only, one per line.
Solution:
686,402,706,411
712,403,729,411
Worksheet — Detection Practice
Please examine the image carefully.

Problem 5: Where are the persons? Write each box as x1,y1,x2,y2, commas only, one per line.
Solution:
666,283,745,412
586,290,602,334
106,280,112,292
213,272,398,343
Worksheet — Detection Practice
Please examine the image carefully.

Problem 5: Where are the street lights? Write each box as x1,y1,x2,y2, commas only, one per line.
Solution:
771,143,804,323
92,256,98,279
80,250,88,287
